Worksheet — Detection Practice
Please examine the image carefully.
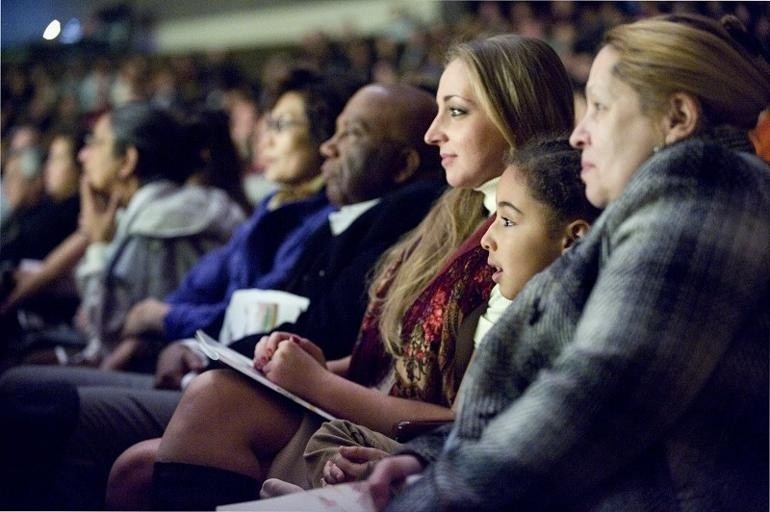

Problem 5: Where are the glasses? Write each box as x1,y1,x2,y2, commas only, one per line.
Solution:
264,113,314,135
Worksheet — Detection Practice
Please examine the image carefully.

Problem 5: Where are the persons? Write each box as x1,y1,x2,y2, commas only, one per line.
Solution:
368,13,770,511
78,99,247,369
0,121,92,347
319,136,606,484
107,34,575,511
0,80,440,510
100,66,348,372
